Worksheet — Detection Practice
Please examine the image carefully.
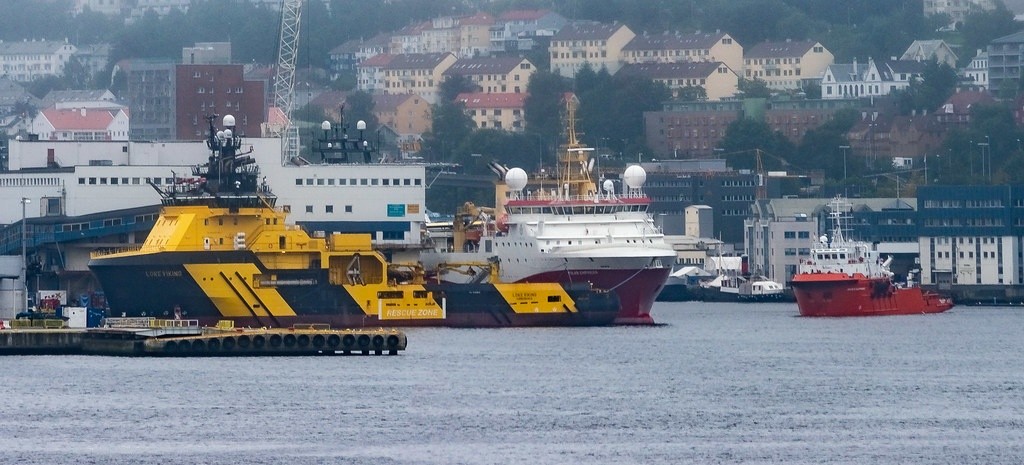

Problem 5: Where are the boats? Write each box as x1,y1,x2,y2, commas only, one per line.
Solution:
87,114,622,330
789,192,955,315
416,89,678,326
655,262,797,303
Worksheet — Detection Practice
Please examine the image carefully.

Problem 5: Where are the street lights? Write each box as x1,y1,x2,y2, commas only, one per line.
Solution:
839,144,849,198
977,142,990,177
20,196,31,312
